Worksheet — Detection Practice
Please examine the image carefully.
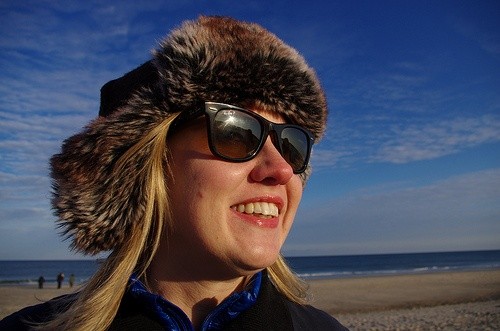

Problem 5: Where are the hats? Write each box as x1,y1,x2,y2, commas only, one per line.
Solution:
49,14,327,256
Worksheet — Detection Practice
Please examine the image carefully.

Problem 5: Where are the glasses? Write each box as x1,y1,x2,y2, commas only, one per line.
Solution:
173,100,315,174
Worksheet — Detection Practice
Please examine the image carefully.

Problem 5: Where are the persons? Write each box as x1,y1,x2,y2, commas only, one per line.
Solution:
56,272,64,289
0,14,348,331
